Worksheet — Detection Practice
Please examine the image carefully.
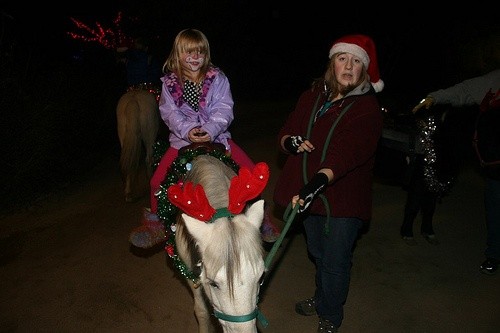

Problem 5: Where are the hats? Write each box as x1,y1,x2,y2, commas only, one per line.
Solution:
329,35,384,92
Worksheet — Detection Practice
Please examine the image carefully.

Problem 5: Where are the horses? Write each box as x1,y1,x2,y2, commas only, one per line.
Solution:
116,83,159,203
164,142,271,333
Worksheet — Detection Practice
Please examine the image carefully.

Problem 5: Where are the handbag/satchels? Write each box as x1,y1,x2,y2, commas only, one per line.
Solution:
468,107,500,180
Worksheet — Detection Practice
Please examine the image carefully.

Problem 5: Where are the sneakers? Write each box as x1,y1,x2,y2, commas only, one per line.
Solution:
318,319,336,333
295,297,317,316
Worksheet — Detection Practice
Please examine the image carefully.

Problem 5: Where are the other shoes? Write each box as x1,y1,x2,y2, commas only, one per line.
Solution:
260,202,281,242
403,235,416,247
421,232,440,246
480,257,500,274
129,214,166,248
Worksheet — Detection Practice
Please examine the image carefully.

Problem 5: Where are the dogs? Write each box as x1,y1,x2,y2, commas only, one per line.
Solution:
372,142,464,246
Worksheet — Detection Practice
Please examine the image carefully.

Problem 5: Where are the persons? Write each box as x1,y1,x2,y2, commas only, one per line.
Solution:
128,28,281,248
279,34,384,333
400,97,460,245
412,69,500,275
